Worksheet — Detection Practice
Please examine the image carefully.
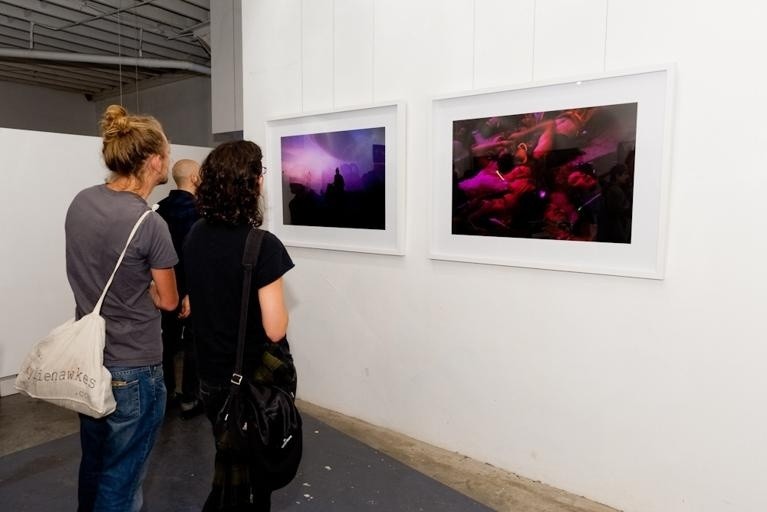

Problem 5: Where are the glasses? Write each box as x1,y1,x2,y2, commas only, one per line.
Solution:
258,167,266,180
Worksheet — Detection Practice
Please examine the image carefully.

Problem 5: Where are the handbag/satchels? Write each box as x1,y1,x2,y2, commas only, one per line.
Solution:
217,379,302,491
13,313,117,419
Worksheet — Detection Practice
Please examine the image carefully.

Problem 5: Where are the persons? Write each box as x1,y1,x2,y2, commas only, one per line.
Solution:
188,141,302,512
64,104,179,512
453,107,635,243
154,159,205,417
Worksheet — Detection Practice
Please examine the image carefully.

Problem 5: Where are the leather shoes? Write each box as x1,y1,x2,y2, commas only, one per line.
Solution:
169,393,203,420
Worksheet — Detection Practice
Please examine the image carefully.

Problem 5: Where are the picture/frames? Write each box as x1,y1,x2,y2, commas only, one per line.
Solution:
263,100,411,260
428,66,673,282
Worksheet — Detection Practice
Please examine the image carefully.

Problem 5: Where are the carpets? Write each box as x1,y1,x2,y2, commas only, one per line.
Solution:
0,408,499,510
